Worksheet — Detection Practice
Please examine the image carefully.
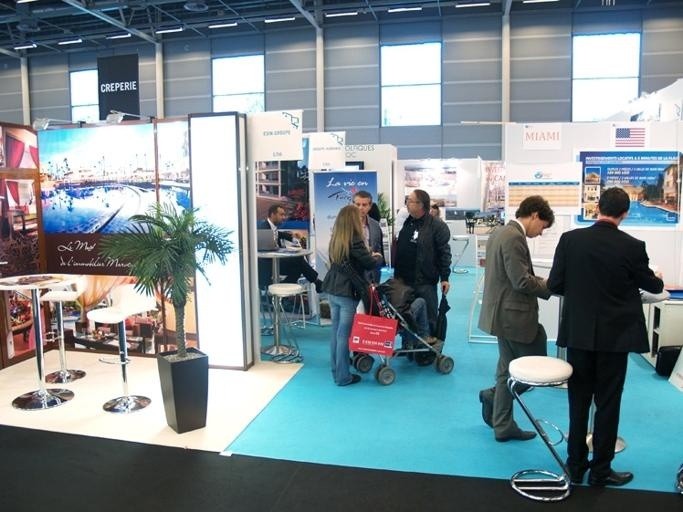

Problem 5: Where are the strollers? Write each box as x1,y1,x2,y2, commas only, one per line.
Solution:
350,269,454,386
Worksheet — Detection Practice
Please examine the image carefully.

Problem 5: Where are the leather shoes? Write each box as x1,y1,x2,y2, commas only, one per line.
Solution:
480,390,536,442
351,374,360,383
418,337,437,344
564,457,633,486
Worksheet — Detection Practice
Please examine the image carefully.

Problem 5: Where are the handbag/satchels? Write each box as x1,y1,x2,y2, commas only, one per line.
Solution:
348,314,398,357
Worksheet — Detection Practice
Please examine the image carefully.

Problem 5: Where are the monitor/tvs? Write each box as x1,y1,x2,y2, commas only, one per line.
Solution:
257,229,279,251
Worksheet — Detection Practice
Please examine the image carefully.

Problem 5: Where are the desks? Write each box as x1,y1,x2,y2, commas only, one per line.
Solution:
257,244,313,359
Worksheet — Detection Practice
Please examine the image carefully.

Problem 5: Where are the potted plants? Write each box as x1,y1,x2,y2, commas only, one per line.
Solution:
95,196,242,434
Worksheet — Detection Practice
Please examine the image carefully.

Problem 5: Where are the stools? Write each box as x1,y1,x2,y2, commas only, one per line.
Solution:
0,271,156,416
451,235,470,274
267,282,303,366
505,354,574,503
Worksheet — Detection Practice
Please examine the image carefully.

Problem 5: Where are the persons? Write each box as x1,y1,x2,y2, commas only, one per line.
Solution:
260,204,324,294
478,194,552,443
546,186,664,489
319,190,453,387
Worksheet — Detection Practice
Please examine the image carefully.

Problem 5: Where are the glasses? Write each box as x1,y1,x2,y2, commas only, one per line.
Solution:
405,195,421,205
